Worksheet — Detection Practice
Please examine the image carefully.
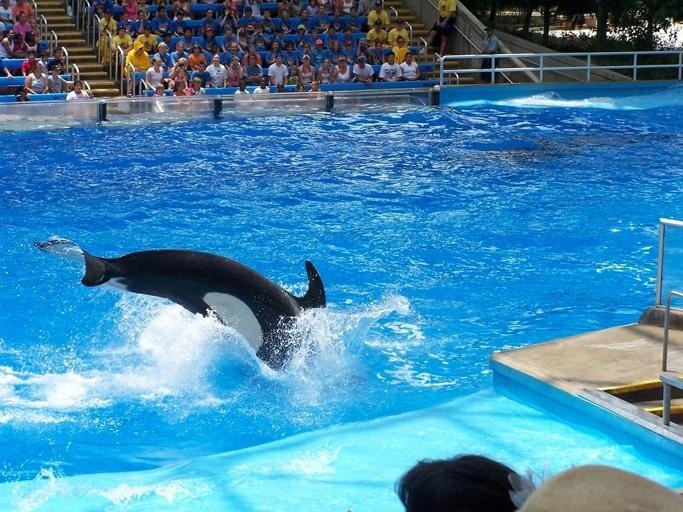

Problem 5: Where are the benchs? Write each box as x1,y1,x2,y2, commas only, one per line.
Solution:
0,53,74,102
85,0,449,99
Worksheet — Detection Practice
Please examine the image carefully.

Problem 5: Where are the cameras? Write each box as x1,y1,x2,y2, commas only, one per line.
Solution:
178,62,185,67
228,11,232,15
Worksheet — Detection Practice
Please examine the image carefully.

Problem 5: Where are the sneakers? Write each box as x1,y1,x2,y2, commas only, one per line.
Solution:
420,48,429,55
436,55,446,62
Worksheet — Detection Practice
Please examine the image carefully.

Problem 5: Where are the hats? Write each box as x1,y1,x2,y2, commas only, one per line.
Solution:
315,39,324,46
297,24,306,32
104,8,111,13
158,42,168,48
484,25,494,30
396,18,404,24
374,20,381,24
245,6,251,11
375,1,381,5
144,24,152,29
302,55,310,60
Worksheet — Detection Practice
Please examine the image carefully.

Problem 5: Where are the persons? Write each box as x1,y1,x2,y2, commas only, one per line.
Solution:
419,0,457,61
393,452,683,512
478,25,500,83
0,0,422,114
